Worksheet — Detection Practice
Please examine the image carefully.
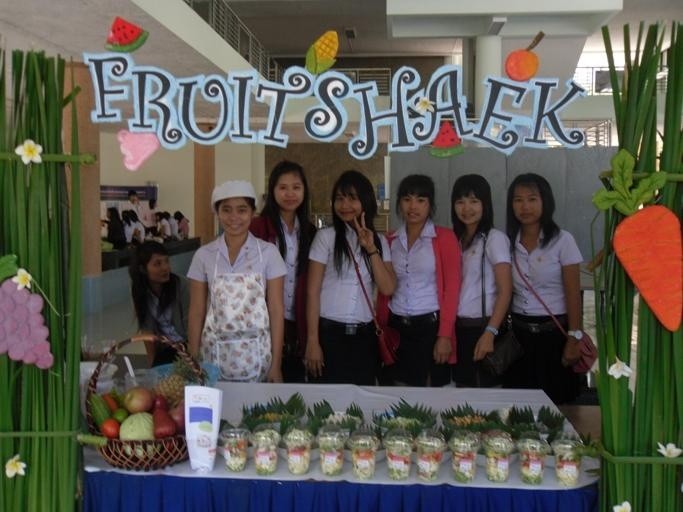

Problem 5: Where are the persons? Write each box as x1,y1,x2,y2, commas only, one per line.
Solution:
245,160,316,385
384,174,462,385
183,180,287,384
98,186,192,264
127,240,190,366
302,170,397,385
451,174,512,388
505,173,585,400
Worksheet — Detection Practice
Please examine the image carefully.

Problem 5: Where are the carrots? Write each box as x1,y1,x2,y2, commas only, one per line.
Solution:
590,148,682,332
102,395,120,413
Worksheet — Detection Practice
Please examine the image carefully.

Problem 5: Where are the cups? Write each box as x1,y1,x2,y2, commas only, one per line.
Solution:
282,432,316,475
249,430,282,475
415,430,448,482
482,430,516,483
381,428,415,481
449,429,481,483
347,428,380,479
314,424,349,476
551,439,583,485
217,428,251,473
517,430,551,484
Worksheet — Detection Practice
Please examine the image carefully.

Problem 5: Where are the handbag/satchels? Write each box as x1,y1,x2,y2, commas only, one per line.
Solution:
483,330,519,382
376,326,396,364
572,333,597,372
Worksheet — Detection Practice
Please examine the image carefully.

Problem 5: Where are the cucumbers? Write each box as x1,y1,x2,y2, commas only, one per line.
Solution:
89,393,112,432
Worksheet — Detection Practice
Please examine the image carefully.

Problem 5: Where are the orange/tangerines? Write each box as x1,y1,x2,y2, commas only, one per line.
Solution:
504,29,547,83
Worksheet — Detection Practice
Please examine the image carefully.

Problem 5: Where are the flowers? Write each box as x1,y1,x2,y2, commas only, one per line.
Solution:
607,354,632,382
657,440,681,461
13,136,44,167
3,454,28,482
613,500,631,512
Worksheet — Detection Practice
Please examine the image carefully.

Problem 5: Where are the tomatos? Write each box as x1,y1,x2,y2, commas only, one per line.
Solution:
98,417,121,440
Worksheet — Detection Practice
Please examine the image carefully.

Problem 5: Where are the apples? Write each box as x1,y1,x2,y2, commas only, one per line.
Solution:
152,394,168,412
153,410,171,424
122,386,153,414
152,415,177,439
168,399,186,429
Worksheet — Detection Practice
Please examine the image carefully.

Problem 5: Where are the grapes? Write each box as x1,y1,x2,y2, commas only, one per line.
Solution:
0,275,56,370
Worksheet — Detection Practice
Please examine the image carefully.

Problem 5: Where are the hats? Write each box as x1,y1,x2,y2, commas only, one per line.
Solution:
210,181,258,212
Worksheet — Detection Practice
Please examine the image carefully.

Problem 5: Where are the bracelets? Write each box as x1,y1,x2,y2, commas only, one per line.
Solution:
485,325,499,337
366,249,380,256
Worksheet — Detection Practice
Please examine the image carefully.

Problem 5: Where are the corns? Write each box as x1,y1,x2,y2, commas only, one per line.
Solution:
304,30,340,77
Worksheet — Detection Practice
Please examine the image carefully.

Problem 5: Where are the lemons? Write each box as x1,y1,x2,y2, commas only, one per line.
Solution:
112,408,128,424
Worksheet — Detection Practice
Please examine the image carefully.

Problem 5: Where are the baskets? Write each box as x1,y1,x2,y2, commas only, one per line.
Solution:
85,335,205,472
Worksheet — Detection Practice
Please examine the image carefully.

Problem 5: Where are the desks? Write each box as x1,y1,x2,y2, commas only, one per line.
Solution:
79,382,601,512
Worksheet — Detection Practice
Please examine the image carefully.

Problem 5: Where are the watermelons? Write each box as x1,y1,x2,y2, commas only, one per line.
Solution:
429,120,464,158
103,16,150,54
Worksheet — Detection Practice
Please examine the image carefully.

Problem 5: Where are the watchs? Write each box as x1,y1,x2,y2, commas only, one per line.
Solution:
567,328,583,340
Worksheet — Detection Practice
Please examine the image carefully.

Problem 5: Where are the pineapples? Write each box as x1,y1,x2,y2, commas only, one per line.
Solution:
149,356,196,408
